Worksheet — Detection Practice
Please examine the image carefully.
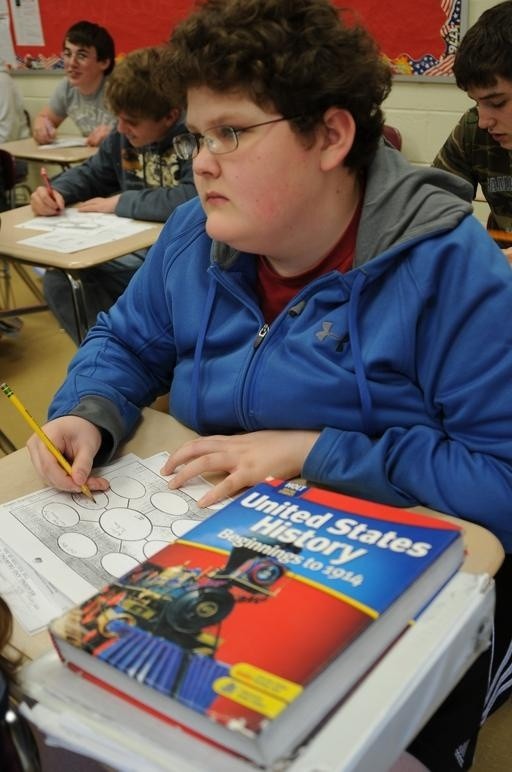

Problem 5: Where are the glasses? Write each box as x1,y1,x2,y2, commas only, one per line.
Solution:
63,49,99,62
172,112,303,162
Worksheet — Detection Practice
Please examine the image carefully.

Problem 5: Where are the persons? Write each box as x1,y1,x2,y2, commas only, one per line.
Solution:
0,66,29,206
31,44,193,349
23,0,512,535
31,19,120,150
429,0,512,257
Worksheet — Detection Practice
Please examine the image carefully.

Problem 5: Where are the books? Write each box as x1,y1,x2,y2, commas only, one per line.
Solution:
49,473,466,772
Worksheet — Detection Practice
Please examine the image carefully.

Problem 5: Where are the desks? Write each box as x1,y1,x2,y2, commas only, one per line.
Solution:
0,406,505,772
0,134,101,172
0,202,166,346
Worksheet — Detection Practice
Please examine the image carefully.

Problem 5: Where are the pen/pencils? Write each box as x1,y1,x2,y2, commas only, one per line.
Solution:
40,167,62,215
1,381,95,500
